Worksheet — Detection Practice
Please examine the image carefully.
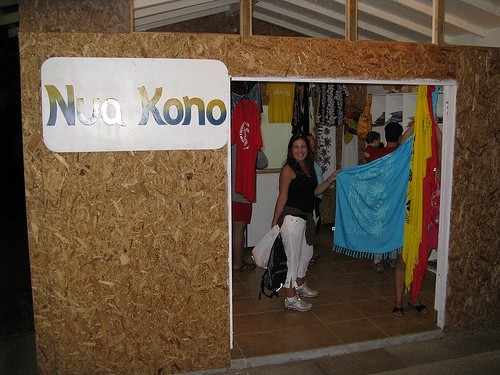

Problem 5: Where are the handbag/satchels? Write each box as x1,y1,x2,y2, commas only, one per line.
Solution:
251,225,281,270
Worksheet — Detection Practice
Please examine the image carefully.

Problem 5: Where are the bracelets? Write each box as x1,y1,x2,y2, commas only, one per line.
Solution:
326,178,332,185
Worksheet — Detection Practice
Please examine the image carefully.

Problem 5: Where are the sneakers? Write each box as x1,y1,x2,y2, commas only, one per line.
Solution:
295,284,319,298
309,254,320,265
284,295,312,312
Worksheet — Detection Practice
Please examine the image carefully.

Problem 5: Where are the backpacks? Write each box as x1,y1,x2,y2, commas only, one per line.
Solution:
258,233,287,300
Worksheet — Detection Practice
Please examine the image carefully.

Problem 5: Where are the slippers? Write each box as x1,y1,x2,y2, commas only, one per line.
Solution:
233,260,256,272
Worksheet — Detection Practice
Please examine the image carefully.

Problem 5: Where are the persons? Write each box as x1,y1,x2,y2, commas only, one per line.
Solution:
307,133,324,266
364,123,404,273
271,134,342,312
232,143,269,272
365,131,380,164
393,123,443,317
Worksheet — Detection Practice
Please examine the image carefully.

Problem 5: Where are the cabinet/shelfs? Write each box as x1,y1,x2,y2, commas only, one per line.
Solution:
359,92,443,274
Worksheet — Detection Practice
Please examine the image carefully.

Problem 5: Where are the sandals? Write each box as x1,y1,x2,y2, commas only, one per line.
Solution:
408,298,428,314
392,306,404,317
